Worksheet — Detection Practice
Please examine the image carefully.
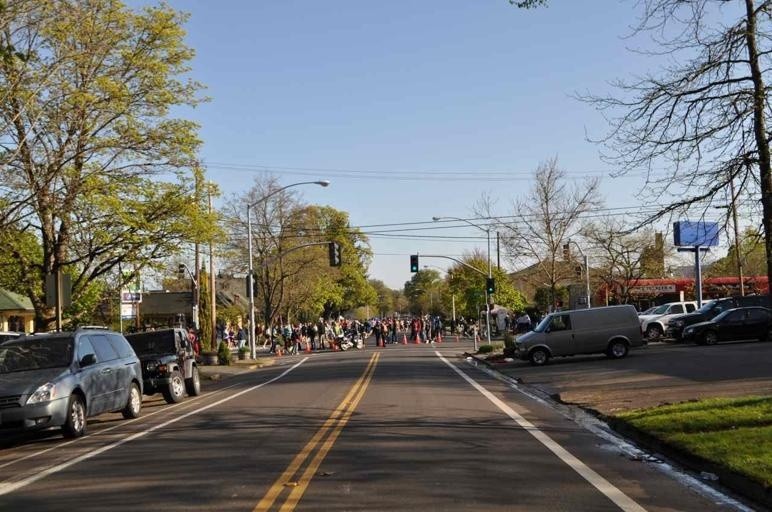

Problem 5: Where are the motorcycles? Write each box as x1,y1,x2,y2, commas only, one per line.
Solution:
335,328,365,351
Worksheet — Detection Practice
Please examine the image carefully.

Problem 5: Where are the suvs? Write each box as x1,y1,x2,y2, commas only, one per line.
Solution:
125,327,201,404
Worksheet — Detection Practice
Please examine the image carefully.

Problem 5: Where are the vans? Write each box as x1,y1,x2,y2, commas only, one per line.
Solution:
512,304,643,367
2,326,146,440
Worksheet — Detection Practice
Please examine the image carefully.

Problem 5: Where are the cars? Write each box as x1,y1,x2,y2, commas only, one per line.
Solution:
639,296,772,345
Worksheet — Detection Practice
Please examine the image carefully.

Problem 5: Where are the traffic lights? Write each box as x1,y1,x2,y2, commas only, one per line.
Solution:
486,277,495,293
330,242,340,266
411,255,419,273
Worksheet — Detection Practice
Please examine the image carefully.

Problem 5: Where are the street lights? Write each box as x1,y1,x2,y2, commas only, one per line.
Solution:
433,216,492,346
178,262,201,304
245,180,328,357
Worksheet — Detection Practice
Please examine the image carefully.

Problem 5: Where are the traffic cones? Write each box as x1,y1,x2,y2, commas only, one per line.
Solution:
416,334,420,344
437,332,442,342
378,338,383,347
305,342,311,353
402,334,407,344
